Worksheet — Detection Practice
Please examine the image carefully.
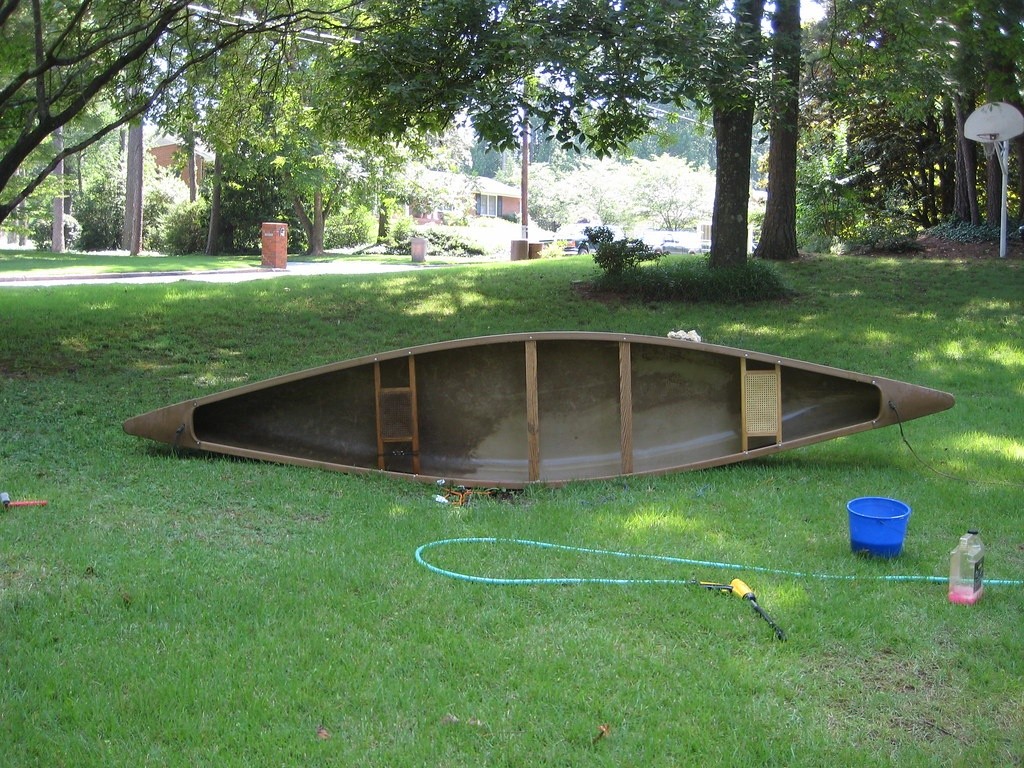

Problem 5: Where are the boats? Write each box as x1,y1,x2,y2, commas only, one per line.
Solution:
122,330,958,491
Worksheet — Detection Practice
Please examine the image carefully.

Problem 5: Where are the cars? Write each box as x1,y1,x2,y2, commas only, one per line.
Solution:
540,218,713,256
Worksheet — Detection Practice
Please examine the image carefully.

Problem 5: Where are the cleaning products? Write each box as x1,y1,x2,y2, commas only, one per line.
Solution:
948,529,986,605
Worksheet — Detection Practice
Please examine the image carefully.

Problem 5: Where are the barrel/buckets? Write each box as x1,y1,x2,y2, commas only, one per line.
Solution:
948,530,984,605
846,496,911,560
